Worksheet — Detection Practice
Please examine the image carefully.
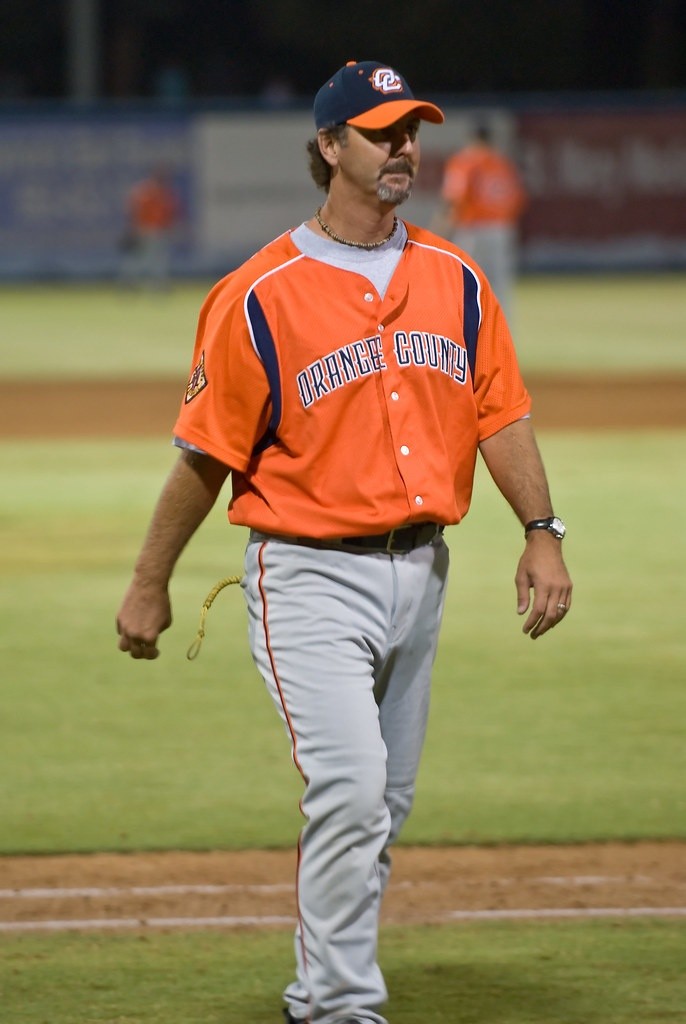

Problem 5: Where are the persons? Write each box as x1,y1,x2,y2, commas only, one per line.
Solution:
430,113,524,306
128,165,181,277
115,57,574,1023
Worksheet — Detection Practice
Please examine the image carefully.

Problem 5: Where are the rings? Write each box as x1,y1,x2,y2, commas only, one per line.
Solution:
557,604,566,609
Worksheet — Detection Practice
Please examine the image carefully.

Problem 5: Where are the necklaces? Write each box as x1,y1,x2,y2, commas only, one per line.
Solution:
315,206,398,249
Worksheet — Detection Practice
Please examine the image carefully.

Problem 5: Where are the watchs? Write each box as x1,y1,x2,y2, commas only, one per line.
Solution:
524,516,566,540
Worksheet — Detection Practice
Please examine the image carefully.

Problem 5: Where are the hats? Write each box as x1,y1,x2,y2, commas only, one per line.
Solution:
313,61,445,133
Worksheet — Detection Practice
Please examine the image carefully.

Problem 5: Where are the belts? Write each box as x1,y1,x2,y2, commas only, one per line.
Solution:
295,522,446,554
